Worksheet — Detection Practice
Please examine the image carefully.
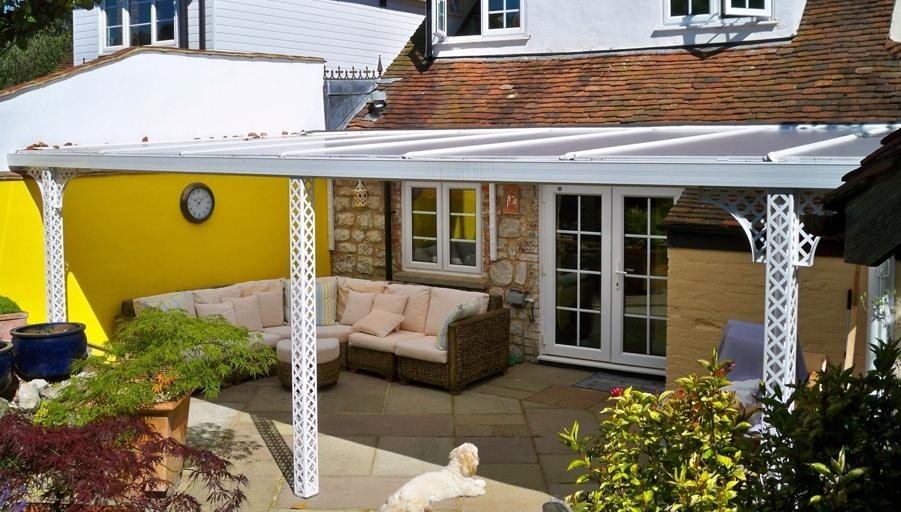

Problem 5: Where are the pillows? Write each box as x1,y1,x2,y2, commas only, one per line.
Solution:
219,294,263,330
335,289,375,326
249,286,286,327
373,293,410,332
196,299,232,336
434,296,479,348
279,277,337,329
353,308,406,336
144,289,198,322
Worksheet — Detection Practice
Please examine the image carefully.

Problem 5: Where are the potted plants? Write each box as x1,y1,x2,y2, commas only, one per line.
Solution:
1,294,31,344
31,309,281,502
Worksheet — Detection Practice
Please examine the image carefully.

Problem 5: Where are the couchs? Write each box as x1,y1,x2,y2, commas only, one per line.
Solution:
120,269,511,399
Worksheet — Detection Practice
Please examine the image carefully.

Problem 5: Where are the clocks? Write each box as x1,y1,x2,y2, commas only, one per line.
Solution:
176,181,218,225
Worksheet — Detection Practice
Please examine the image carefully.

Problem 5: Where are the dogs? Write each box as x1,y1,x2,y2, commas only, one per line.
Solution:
381,441,488,512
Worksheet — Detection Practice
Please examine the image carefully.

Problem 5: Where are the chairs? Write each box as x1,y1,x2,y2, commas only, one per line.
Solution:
271,336,344,391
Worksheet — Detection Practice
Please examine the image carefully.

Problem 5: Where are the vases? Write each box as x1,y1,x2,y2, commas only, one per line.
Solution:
0,337,20,395
9,319,90,385
